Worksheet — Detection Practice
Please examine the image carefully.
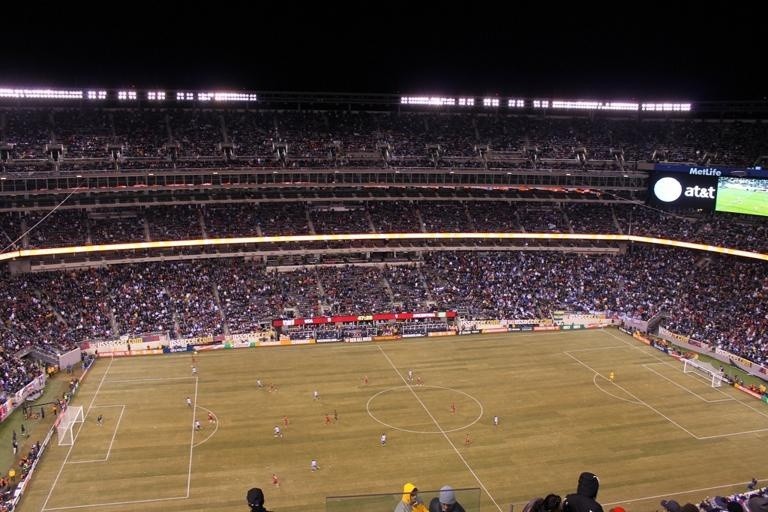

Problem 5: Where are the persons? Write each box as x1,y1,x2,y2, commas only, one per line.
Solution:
191,366,199,376
464,433,471,446
521,470,767,512
97,414,104,426
493,414,499,425
0,351,98,512
393,481,431,512
312,389,321,401
608,369,614,382
273,425,283,438
672,351,768,396
408,369,424,387
0,99,768,351
428,486,467,512
380,432,387,447
325,413,333,425
207,411,215,423
185,396,192,408
246,487,269,511
332,409,339,421
282,414,289,429
310,458,320,472
272,473,280,488
255,378,263,389
194,419,202,432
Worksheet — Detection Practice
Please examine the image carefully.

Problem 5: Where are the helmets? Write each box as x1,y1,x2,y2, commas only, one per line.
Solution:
577,472,599,497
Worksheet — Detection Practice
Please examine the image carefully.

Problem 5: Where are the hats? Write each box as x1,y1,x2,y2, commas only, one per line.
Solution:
439,485,457,504
661,487,768,512
246,487,265,508
